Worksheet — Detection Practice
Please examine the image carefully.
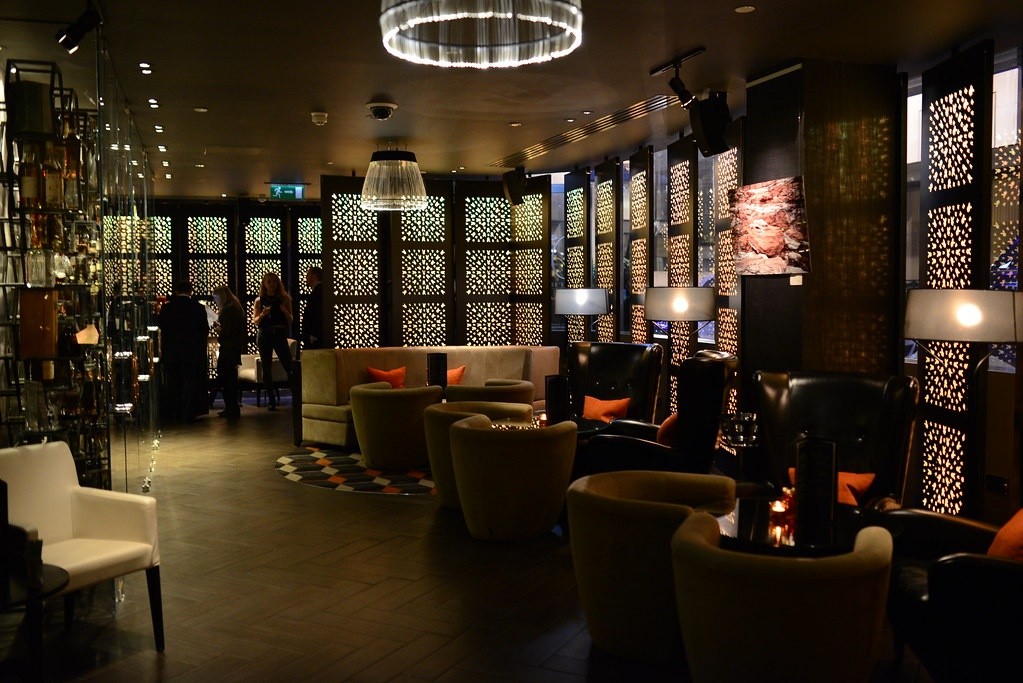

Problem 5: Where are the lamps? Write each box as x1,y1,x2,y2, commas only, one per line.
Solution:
549,288,611,316
643,288,719,321
378,1,585,71
898,289,1023,345
361,140,431,216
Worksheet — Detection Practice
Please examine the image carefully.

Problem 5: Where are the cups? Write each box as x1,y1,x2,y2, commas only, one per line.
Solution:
263,305,272,313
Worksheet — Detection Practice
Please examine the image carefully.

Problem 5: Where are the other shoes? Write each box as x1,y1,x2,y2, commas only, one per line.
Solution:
217,408,240,417
185,418,203,424
267,394,276,410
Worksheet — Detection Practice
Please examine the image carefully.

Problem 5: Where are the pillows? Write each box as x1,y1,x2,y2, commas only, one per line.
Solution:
423,365,467,388
655,412,680,448
582,393,631,427
979,511,1021,563
784,464,878,509
368,365,409,390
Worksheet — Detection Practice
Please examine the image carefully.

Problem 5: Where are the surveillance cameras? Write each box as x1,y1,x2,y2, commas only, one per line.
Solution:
367,103,398,121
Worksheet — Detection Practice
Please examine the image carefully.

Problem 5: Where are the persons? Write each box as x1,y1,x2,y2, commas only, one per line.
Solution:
252,272,296,411
106,278,153,420
303,267,336,351
157,280,208,424
210,286,245,417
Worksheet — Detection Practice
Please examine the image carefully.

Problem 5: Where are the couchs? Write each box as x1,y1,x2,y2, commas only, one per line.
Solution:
301,346,1023,683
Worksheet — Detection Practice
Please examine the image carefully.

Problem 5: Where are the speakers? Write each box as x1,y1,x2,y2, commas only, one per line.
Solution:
502,170,523,206
689,98,729,157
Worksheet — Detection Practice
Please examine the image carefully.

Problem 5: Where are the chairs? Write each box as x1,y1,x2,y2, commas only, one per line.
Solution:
240,337,300,409
0,442,167,653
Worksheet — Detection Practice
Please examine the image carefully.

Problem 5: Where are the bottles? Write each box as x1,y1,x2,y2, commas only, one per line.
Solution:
10,300,109,491
21,96,99,211
26,214,100,285
